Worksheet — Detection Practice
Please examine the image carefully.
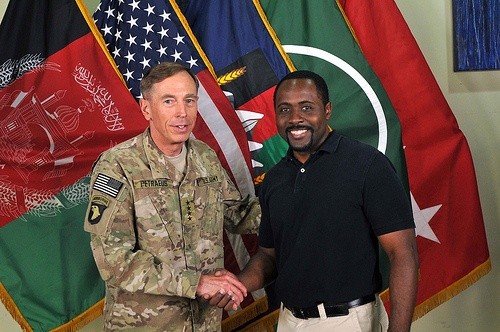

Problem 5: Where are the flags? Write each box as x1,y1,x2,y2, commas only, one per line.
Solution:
343,1,492,323
259,0,418,304
178,0,333,327
0,0,149,332
92,0,280,330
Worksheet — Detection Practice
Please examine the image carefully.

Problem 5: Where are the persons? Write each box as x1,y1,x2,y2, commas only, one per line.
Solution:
204,70,418,332
83,64,264,332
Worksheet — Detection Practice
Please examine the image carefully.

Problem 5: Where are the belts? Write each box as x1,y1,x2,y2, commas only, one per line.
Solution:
283,294,376,319
